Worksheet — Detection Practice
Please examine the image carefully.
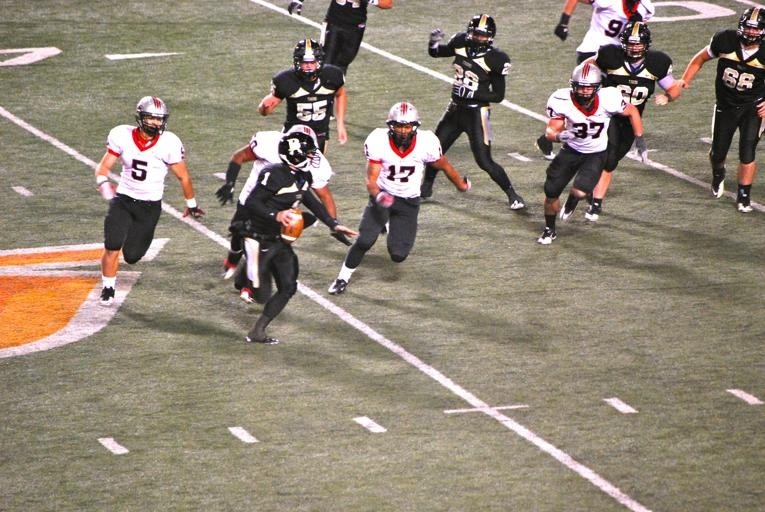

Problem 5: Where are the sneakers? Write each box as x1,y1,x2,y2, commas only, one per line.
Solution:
99,287,114,306
737,189,753,212
712,165,727,198
220,260,347,344
417,136,603,245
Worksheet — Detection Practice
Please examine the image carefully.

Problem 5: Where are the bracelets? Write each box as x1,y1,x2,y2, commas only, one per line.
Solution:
664,93,673,103
680,8,765,214
184,198,197,208
95,175,107,185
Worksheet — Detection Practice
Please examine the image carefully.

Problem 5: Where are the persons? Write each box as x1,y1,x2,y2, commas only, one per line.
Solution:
258,39,348,155
288,0,393,74
536,62,647,246
582,19,682,222
535,0,655,160
95,96,205,305
328,102,472,295
235,132,360,346
417,14,526,210
215,125,351,280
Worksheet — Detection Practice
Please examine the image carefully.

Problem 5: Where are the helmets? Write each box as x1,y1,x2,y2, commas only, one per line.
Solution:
465,13,496,59
569,64,602,105
618,21,652,62
737,7,765,46
386,102,420,143
278,38,326,175
134,96,170,135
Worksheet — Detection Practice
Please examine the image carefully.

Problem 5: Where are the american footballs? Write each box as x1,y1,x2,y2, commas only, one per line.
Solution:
280,207,304,245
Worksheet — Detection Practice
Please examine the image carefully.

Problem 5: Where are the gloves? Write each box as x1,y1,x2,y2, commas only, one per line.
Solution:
555,131,570,142
634,149,648,165
215,181,235,206
330,228,352,247
287,0,303,16
430,28,445,44
95,181,118,201
452,83,474,99
376,191,395,209
555,23,568,40
182,206,204,218
